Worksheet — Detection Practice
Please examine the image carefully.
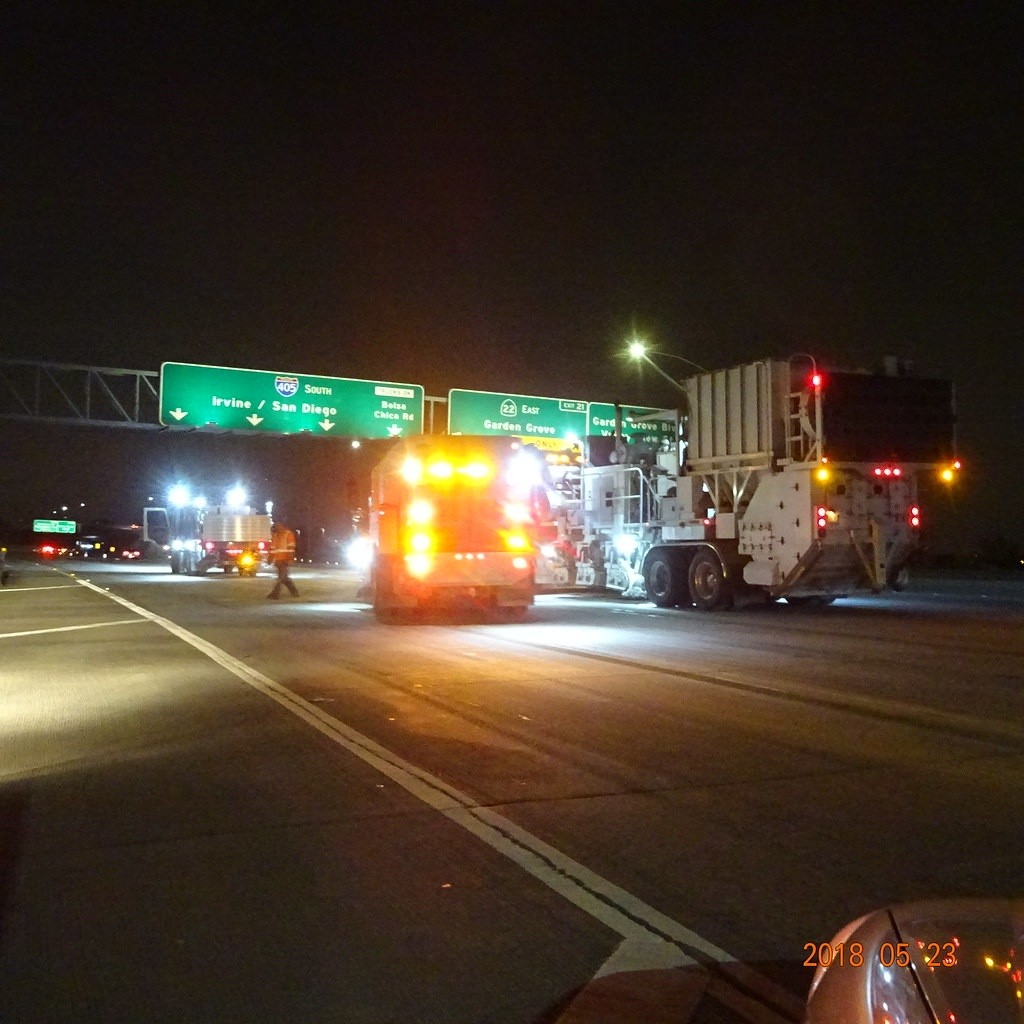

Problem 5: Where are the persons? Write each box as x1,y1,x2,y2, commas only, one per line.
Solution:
266,520,300,599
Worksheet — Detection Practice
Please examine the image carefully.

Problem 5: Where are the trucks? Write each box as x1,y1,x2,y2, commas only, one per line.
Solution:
368,433,546,620
141,481,274,578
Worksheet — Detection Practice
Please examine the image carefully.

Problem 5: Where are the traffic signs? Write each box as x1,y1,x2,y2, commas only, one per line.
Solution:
158,360,426,443
447,387,589,464
587,401,682,467
32,519,76,534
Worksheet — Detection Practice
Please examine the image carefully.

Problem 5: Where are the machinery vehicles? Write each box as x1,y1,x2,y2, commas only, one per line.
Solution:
637,362,922,616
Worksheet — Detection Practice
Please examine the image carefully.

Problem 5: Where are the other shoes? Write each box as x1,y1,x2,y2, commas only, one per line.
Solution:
267,593,279,600
289,591,300,597
